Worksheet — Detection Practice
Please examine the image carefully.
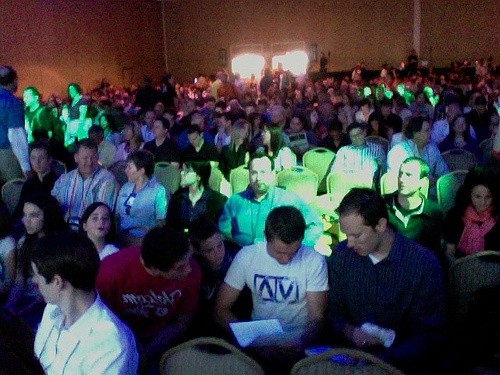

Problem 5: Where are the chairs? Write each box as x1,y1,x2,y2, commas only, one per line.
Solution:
0,66,500,375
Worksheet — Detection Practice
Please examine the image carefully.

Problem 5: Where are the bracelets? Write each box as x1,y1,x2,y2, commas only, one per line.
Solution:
351,326,355,340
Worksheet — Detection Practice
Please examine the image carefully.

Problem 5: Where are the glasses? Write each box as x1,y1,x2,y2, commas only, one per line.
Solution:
179,169,196,174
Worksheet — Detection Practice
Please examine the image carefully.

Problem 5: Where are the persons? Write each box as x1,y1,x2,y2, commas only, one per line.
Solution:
383,157,446,256
212,204,329,375
260,122,294,175
31,128,76,172
12,141,59,238
23,87,52,142
387,116,450,203
438,115,487,171
93,225,204,375
0,66,30,182
113,119,144,164
216,151,324,249
165,159,227,236
326,186,448,375
331,123,388,193
0,198,15,306
191,216,251,356
113,150,169,247
182,125,224,174
6,194,69,334
51,138,121,232
78,202,121,261
45,60,500,129
74,124,117,170
319,51,328,72
141,118,182,169
220,118,255,184
408,49,418,67
30,228,141,375
481,114,500,170
444,180,500,267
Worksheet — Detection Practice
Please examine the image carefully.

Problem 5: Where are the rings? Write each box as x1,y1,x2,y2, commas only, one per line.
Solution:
362,340,366,346
